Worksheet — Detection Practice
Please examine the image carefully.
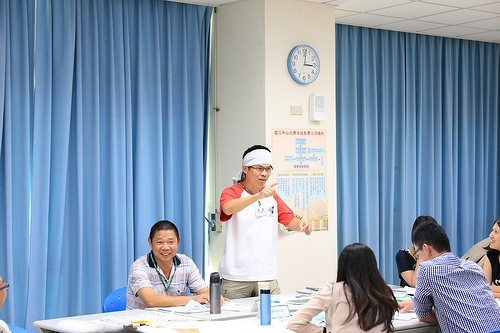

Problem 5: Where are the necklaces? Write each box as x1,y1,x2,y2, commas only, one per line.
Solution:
238,182,253,195
405,246,417,260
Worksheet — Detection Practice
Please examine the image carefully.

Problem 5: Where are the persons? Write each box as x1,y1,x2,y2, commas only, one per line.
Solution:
286,242,400,333
481,219,500,298
215,144,312,302
126,220,231,310
397,222,500,333
0,277,13,333
395,215,439,288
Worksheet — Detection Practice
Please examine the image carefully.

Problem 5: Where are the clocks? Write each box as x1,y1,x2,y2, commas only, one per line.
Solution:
286,44,321,85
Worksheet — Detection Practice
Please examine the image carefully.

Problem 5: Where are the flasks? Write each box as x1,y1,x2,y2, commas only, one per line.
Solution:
260,287,271,324
211,272,221,315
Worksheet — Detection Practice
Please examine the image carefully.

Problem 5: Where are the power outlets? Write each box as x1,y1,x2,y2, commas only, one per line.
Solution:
291,106,303,115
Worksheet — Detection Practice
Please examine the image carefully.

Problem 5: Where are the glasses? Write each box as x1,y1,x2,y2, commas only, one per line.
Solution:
0,284,9,290
251,166,273,173
412,247,422,260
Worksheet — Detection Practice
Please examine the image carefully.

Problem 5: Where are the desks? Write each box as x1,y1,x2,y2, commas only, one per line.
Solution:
34,283,436,333
